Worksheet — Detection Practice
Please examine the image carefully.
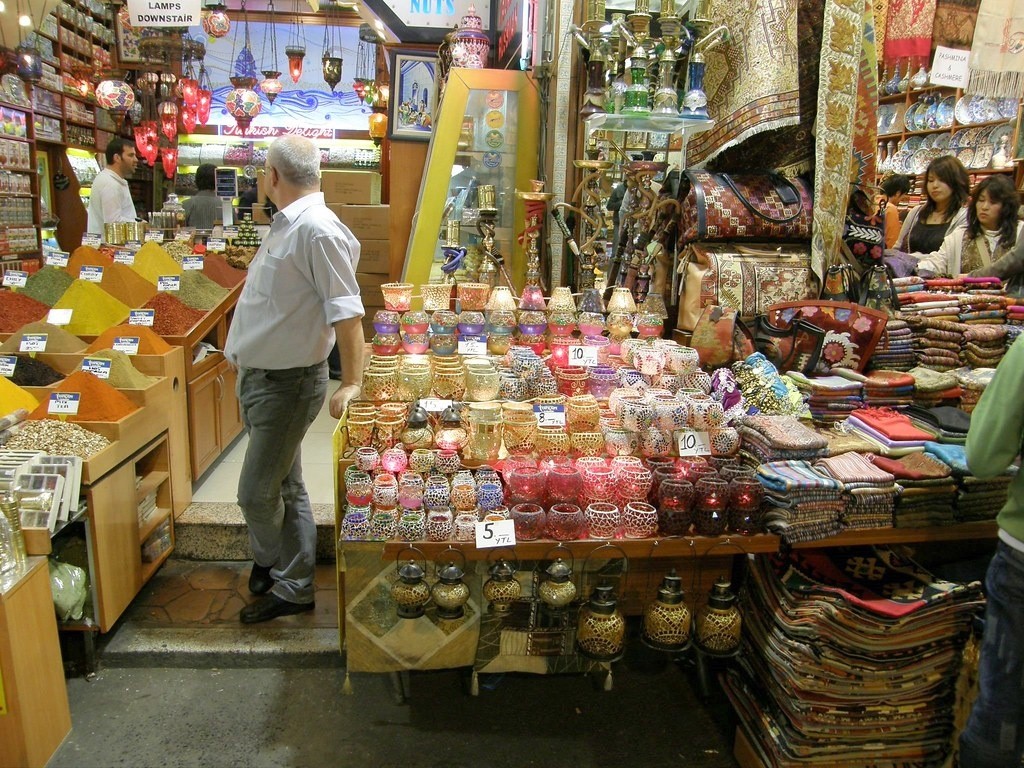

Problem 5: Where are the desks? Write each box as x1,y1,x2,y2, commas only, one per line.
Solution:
383,519,998,560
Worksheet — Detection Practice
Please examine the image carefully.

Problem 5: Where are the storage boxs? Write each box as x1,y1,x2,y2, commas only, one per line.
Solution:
253,170,393,343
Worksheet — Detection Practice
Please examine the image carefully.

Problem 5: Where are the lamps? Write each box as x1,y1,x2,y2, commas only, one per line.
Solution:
368,104,388,147
322,0,343,91
285,0,307,84
260,0,282,106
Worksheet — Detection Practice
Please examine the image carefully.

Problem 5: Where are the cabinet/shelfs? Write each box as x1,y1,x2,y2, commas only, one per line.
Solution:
0,280,250,768
878,72,1024,193
0,0,137,289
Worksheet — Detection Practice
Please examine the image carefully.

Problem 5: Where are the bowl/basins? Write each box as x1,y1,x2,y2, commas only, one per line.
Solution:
451,188,478,207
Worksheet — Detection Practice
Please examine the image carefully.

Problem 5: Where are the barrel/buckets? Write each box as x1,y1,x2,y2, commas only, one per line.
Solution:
104,222,144,244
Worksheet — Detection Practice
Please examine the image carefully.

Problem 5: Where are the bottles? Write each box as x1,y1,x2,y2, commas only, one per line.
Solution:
86,111,94,124
68,126,94,146
148,210,186,239
876,142,901,174
879,59,936,97
234,213,261,246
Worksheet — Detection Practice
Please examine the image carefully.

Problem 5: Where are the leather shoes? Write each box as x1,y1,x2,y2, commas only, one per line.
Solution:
240,591,316,623
248,562,277,594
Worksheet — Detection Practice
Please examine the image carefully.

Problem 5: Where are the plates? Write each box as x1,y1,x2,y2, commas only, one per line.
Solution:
949,123,1015,168
904,96,955,132
955,94,1019,125
891,133,952,175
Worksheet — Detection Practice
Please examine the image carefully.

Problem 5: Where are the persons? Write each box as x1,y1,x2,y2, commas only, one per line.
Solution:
955,222,1024,300
875,174,911,249
890,155,971,257
87,138,138,245
965,330,1024,768
915,173,1024,279
223,134,365,624
182,163,223,229
606,168,635,286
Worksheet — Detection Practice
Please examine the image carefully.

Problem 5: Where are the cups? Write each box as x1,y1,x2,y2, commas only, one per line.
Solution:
478,185,495,209
448,220,459,245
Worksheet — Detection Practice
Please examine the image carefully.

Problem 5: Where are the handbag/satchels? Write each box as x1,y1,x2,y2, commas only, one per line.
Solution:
859,264,901,313
675,167,813,241
821,264,859,305
675,240,828,331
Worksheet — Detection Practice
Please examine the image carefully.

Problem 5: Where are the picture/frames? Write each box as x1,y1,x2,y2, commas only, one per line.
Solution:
388,49,442,143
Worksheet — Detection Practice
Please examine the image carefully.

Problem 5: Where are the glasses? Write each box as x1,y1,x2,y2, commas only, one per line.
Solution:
260,167,272,176
